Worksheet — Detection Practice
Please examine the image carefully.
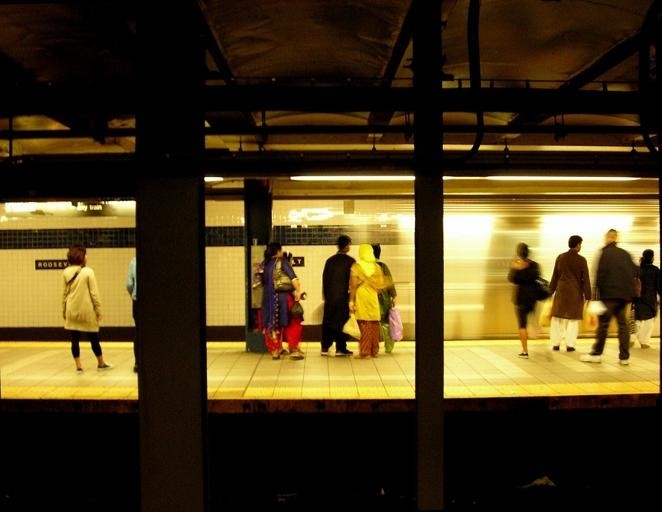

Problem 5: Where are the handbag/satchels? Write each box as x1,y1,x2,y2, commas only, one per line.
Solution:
535,277,550,300
389,306,404,342
274,271,296,293
633,277,642,298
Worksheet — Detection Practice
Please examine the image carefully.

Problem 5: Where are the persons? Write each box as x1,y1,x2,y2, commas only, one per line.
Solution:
124,248,139,374
631,248,661,353
548,234,591,356
249,238,308,361
504,242,542,358
60,244,112,372
318,234,399,359
577,227,640,369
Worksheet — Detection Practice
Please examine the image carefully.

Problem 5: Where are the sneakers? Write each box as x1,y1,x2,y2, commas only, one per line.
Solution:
580,355,601,363
620,359,630,365
518,353,529,358
78,368,82,374
321,349,329,356
553,346,559,350
567,347,575,352
98,364,110,369
336,350,353,356
